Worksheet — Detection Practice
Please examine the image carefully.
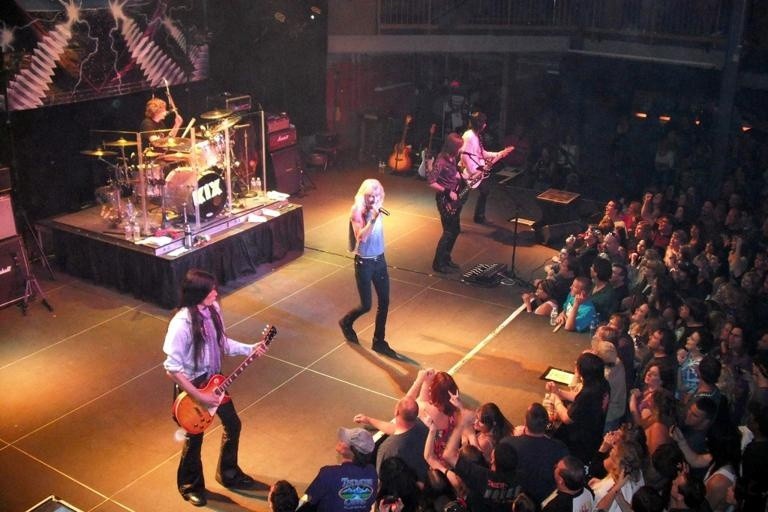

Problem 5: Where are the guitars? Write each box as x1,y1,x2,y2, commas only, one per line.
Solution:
464,145,514,190
389,115,413,171
173,324,278,435
417,124,438,179
440,166,493,220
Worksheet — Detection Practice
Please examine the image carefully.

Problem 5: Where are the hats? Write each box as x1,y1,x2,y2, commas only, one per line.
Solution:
336,427,376,456
591,336,619,365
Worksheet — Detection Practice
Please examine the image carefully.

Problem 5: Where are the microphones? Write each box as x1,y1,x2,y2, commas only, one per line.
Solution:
459,149,476,156
378,207,390,216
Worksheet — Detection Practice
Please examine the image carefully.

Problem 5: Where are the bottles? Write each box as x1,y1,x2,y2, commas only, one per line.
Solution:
133,222,140,240
256,177,262,192
126,198,134,219
184,223,192,250
376,161,386,175
250,178,255,191
124,222,132,240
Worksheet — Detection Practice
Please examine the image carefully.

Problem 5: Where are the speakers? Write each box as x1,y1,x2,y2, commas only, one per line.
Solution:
268,144,305,197
0,233,36,310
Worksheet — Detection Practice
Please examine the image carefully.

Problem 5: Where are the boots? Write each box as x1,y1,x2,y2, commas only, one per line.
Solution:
370,327,396,359
337,309,361,346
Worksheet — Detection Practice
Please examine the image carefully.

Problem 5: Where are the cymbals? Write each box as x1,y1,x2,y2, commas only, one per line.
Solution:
200,109,234,119
103,139,142,146
80,150,118,155
152,135,190,147
211,115,242,134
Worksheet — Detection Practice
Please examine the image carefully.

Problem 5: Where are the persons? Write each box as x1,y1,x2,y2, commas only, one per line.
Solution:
140,98,183,147
164,267,268,507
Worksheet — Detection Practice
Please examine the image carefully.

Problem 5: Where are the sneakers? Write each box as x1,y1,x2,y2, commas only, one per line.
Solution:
432,261,460,274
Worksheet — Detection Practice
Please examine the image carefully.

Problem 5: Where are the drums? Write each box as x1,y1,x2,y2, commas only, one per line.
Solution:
164,166,227,221
132,164,164,197
185,137,227,173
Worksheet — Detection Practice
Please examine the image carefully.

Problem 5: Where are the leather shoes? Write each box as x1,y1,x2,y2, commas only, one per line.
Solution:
221,474,256,489
180,485,208,507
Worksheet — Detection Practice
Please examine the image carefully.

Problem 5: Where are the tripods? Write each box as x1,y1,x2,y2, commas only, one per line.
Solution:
472,158,538,290
17,236,53,316
